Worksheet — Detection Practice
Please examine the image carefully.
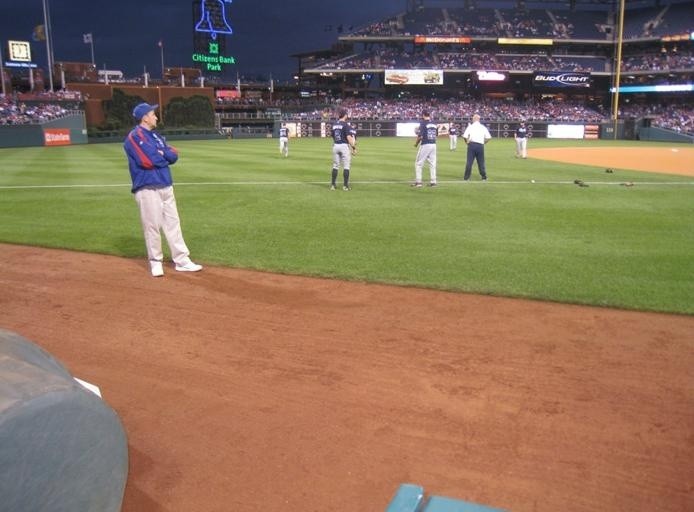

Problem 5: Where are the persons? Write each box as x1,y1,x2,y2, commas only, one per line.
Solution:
0,2,694,154
459,113,493,181
329,110,356,190
512,119,528,159
411,108,438,190
123,101,202,278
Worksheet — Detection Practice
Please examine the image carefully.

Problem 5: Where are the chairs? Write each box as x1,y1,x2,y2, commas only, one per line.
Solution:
1,89,85,126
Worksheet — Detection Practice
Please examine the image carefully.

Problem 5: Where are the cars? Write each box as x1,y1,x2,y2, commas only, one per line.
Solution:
386,74,408,84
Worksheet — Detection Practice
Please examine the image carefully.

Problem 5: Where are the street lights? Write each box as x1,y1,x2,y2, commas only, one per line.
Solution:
156,39,165,80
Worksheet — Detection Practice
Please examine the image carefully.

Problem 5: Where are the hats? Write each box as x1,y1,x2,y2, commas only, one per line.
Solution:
133,102,160,121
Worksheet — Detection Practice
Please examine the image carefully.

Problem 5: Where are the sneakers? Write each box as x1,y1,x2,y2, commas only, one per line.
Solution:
150,261,165,277
482,176,488,180
343,185,352,190
464,178,471,181
431,183,439,187
330,185,337,190
176,262,203,272
411,182,423,188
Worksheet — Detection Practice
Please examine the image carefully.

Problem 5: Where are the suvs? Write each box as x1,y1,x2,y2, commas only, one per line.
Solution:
422,72,440,83
